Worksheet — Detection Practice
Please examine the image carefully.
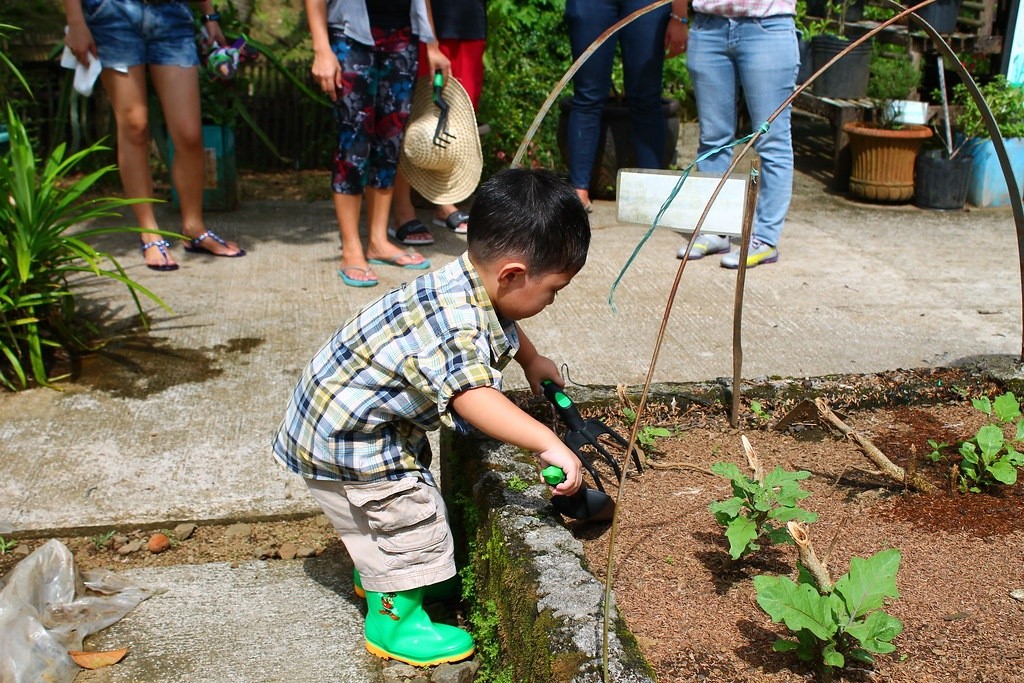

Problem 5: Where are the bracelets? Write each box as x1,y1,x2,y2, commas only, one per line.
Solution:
670,12,688,24
200,13,218,24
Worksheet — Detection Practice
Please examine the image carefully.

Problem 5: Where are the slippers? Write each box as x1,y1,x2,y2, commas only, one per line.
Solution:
366,246,431,270
432,207,470,233
338,260,378,287
387,219,434,244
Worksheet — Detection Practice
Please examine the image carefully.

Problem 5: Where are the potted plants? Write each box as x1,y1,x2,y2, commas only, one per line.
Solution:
557,52,694,200
794,0,1024,210
0,99,193,392
164,0,335,211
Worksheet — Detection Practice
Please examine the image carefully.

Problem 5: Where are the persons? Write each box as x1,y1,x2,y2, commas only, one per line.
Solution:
561,0,688,213
273,165,585,665
305,1,487,287
676,0,801,268
63,0,246,271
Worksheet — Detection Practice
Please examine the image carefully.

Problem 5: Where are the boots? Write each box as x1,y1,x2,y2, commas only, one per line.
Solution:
353,563,462,600
364,585,475,667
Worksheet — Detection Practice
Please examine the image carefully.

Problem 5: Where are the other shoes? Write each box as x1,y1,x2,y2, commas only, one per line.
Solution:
576,199,594,213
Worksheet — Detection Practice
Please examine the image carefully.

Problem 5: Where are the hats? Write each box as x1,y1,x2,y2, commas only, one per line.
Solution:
396,70,484,205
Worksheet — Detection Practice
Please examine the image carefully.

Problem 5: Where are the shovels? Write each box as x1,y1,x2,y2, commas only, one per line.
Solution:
541,466,617,521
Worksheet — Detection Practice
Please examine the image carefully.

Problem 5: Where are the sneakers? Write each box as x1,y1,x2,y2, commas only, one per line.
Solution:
677,230,730,260
720,237,778,269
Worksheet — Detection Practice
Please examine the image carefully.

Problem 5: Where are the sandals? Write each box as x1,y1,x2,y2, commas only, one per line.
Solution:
140,237,179,270
180,226,246,257
